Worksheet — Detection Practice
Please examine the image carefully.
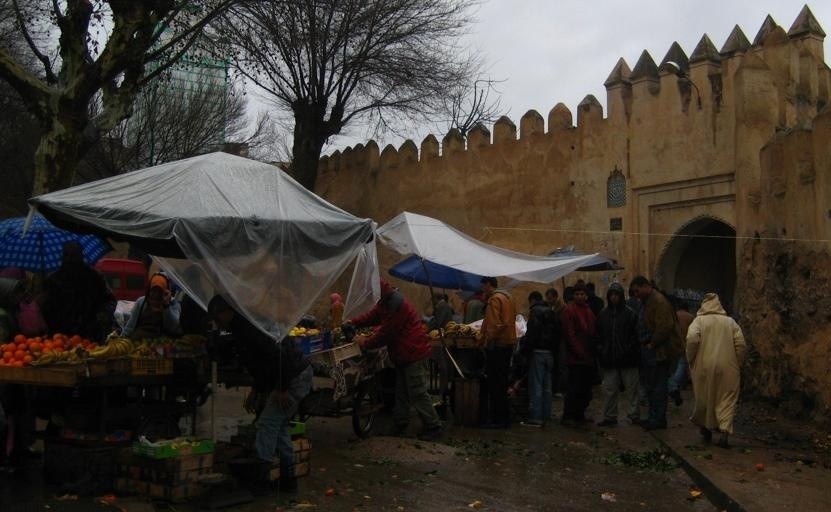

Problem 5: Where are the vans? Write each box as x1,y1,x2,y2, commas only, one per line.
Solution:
97,259,147,301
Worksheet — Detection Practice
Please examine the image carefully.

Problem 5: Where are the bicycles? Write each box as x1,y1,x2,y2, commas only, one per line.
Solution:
350,346,396,439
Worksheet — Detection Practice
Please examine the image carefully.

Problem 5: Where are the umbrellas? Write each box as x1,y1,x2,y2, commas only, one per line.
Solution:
546,248,622,290
0,215,113,272
389,257,489,297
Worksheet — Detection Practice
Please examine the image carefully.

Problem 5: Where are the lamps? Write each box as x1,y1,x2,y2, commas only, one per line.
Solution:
664,61,702,114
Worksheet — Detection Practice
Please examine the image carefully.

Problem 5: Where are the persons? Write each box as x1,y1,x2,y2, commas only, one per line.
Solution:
198,293,315,495
35,238,116,340
120,273,208,338
10,277,42,337
683,290,749,450
329,275,695,442
